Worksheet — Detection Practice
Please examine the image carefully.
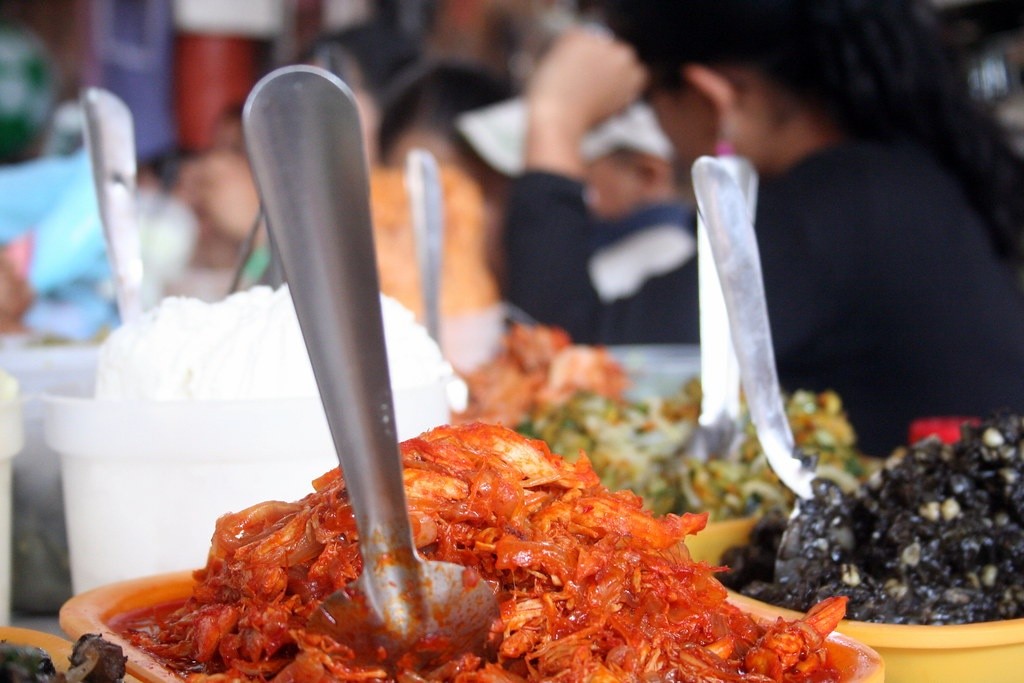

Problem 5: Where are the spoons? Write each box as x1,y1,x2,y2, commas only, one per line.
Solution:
240,68,495,675
688,155,854,589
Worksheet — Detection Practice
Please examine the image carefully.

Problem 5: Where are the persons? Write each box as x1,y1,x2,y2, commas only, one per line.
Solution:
0,0,1024,460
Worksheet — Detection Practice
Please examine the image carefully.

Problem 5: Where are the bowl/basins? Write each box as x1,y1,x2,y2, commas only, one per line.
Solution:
683,513,1024,683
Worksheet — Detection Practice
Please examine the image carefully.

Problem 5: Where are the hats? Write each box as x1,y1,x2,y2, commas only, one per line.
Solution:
454,90,676,187
